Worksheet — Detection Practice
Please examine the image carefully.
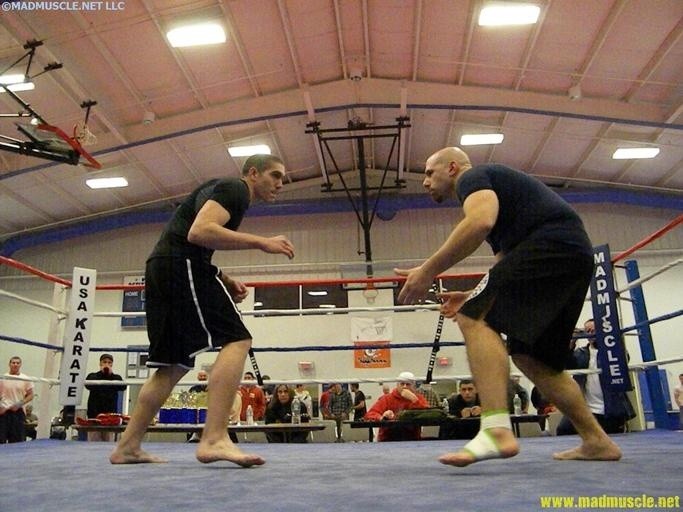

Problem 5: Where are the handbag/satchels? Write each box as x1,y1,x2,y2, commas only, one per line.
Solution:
399,407,446,419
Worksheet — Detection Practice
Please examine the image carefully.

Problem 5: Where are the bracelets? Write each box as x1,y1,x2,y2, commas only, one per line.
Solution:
15,400,23,408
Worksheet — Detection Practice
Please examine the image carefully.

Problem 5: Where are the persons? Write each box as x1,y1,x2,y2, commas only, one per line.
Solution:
106,152,296,469
84,353,127,419
672,372,683,430
190,367,554,444
22,403,38,440
391,146,624,468
0,354,34,443
554,317,637,436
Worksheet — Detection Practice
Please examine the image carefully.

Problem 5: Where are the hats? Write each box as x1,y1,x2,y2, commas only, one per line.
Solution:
396,372,414,384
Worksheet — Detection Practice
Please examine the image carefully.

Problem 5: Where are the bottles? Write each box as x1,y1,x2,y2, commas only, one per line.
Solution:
290,396,300,424
246,405,253,425
442,398,449,417
512,394,521,416
